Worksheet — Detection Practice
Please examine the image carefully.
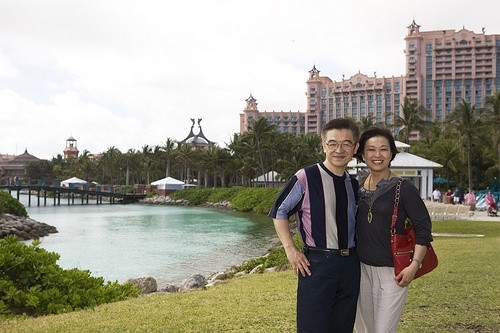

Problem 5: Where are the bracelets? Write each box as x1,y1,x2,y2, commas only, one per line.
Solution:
413,257,423,269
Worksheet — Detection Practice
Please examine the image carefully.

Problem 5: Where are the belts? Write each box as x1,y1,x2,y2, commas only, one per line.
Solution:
303,246,357,258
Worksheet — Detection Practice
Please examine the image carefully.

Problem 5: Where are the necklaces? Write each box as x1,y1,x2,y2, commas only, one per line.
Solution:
367,170,391,223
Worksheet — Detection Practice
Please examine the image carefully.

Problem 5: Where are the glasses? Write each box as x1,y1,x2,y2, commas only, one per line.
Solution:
324,140,357,152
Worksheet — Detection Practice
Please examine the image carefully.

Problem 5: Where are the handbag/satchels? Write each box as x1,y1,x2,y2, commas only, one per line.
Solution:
389,177,439,285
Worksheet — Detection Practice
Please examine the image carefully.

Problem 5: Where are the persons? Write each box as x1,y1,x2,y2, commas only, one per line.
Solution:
464,187,476,211
354,127,434,333
485,192,496,210
266,116,362,333
433,187,441,202
445,187,462,205
